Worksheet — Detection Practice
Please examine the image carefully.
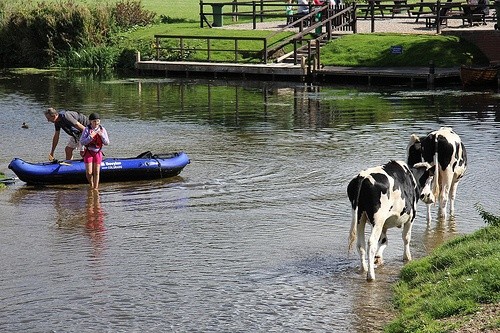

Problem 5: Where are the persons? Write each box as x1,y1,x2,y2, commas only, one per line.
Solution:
80,112,109,190
286,0,342,22
44,107,89,160
465,0,490,15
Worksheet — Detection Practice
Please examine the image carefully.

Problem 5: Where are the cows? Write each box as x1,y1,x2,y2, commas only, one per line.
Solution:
407,127,468,221
346,160,436,283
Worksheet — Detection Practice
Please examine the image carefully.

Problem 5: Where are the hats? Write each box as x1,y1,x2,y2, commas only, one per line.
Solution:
89,113,100,120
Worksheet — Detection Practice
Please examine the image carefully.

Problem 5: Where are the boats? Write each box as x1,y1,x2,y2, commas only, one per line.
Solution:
7,148,191,184
